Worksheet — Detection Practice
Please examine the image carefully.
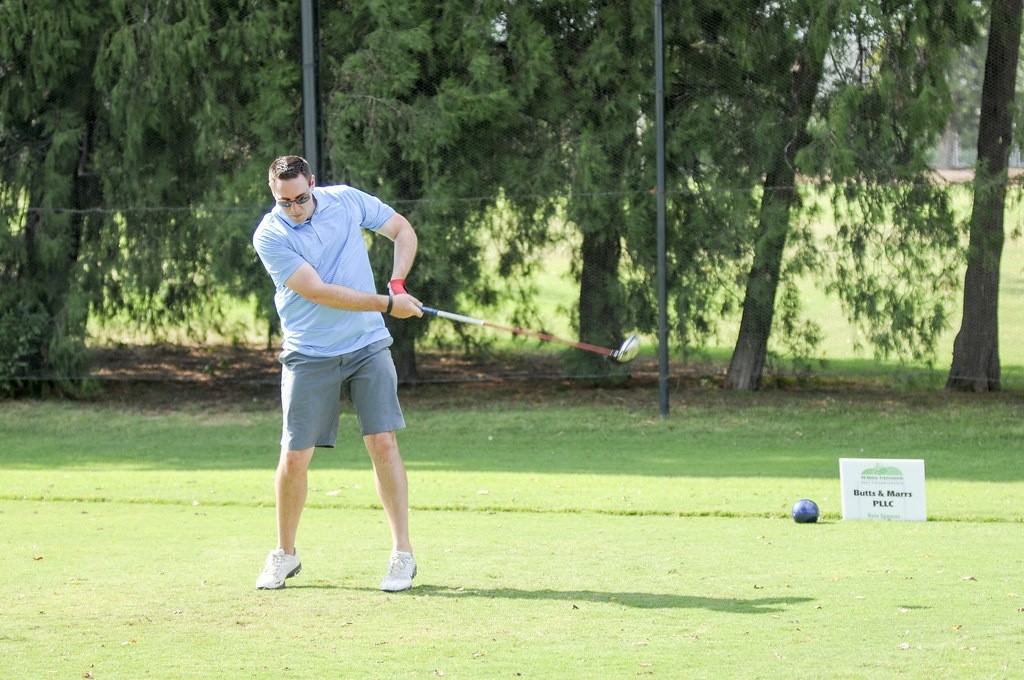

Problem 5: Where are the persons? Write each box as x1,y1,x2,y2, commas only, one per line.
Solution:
254,155,423,592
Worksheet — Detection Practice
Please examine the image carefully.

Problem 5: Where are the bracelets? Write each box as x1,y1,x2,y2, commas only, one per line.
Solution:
385,296,393,315
387,279,408,295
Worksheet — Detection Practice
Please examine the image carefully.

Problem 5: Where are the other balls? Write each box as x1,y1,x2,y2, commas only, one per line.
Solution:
791,500,819,523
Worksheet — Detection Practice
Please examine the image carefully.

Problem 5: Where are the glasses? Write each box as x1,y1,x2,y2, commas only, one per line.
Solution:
272,185,312,208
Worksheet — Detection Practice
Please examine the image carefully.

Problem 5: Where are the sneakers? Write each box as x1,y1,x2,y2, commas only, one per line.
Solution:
381,552,418,593
254,544,303,592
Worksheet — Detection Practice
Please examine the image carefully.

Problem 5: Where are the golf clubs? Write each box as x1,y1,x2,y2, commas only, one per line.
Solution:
423,305,640,364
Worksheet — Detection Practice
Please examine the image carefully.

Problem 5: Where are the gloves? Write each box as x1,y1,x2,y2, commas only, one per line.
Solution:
387,278,409,297
